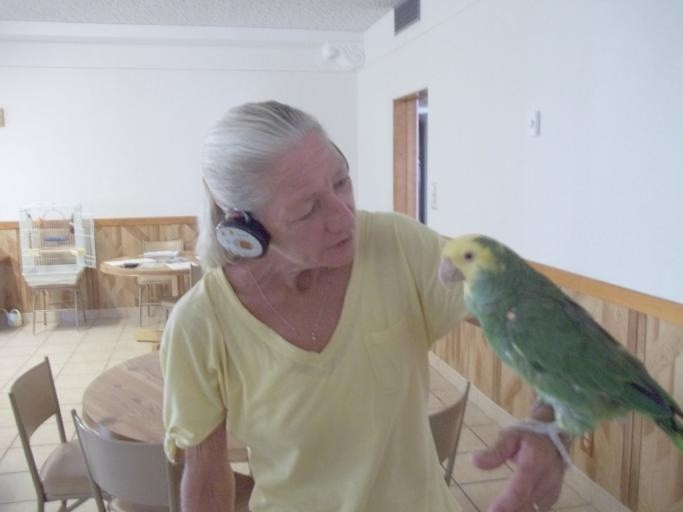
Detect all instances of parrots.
[438,234,683,473]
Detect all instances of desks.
[77,348,248,511]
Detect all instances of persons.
[155,100,582,512]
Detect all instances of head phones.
[214,140,349,261]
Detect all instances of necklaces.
[243,259,341,343]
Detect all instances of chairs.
[427,382,473,482]
[68,405,179,512]
[0,240,212,346]
[8,356,116,512]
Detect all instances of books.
[143,249,179,263]
